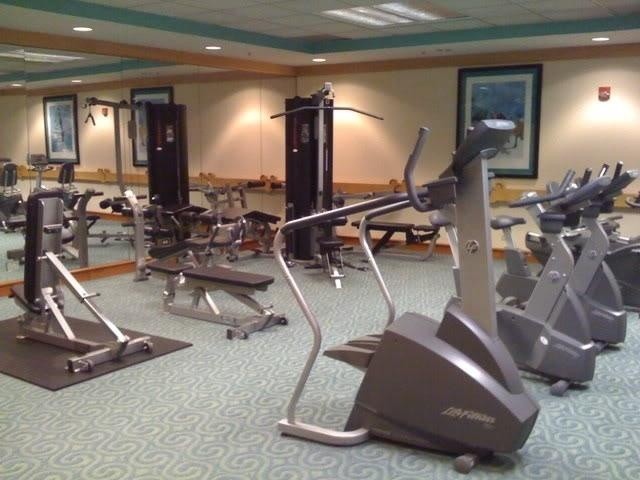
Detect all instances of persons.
[512,113,524,148]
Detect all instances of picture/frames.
[130,86,173,166]
[456,64,542,178]
[42,95,80,165]
